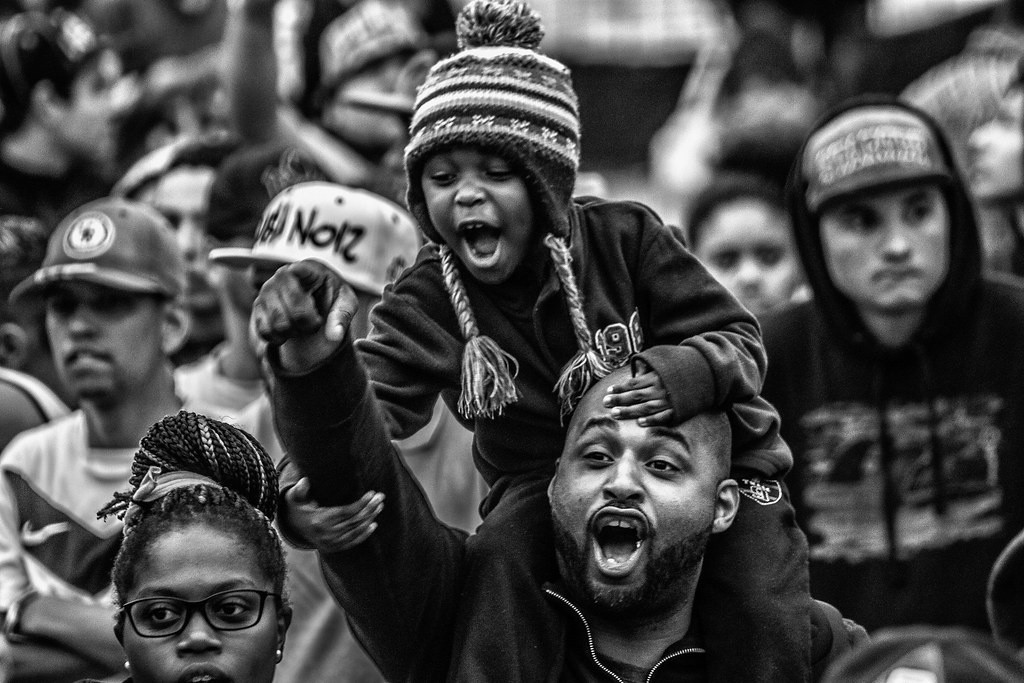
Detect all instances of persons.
[0,0,1024,683]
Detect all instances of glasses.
[116,589,280,639]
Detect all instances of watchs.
[3,590,37,644]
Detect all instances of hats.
[802,105,950,209]
[9,197,185,318]
[405,0,615,419]
[0,8,112,101]
[207,181,423,297]
